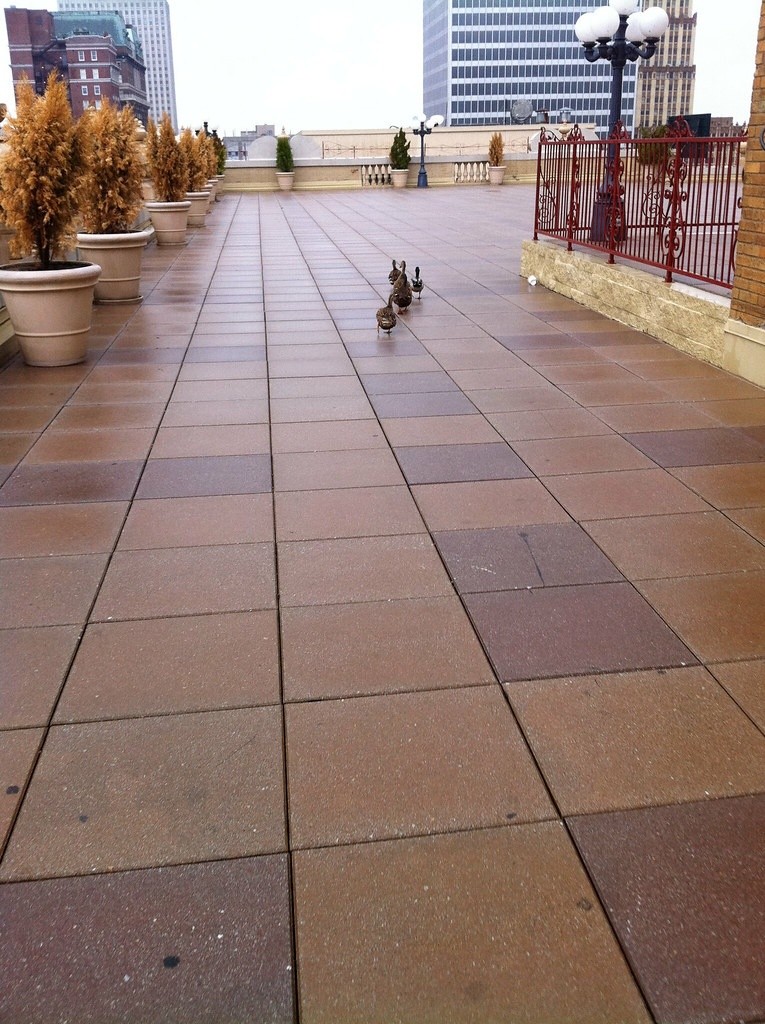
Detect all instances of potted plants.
[0,67,226,367]
[637,123,672,184]
[389,128,411,187]
[275,137,295,190]
[488,133,506,185]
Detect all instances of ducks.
[376,293,400,334]
[392,261,412,314]
[410,267,424,300]
[389,260,402,285]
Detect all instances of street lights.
[410,113,437,187]
[572,0,670,239]
